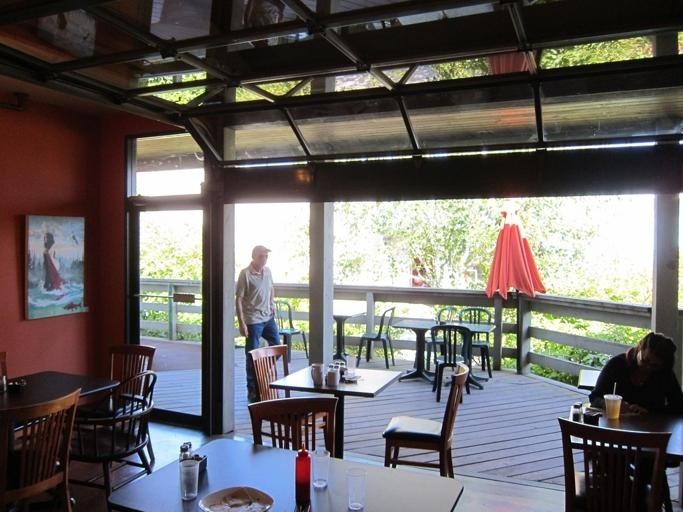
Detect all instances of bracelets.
[270,303,274,307]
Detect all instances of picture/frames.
[20,209,91,322]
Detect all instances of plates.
[197,486,274,512]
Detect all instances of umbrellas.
[484,202,547,301]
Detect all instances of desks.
[312,307,368,364]
[106,437,576,510]
[267,362,403,459]
[564,401,682,491]
[2,368,123,419]
[0,387,84,511]
[573,365,600,392]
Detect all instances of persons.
[588,332,683,415]
[236,245,281,402]
[43,232,65,290]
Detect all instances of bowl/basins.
[5,379,30,392]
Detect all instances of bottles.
[329,360,346,380]
[573,402,582,422]
[1,376,6,393]
[295,444,312,507]
[178,441,192,459]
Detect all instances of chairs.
[243,394,339,459]
[268,299,310,362]
[61,368,160,501]
[353,306,398,370]
[246,343,331,450]
[105,340,157,470]
[386,306,498,406]
[379,361,471,482]
[554,415,675,511]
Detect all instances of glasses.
[641,353,656,367]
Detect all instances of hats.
[252,245,271,254]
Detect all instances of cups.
[346,468,367,511]
[603,393,622,420]
[310,363,324,386]
[178,459,200,502]
[328,369,341,387]
[312,450,330,488]
